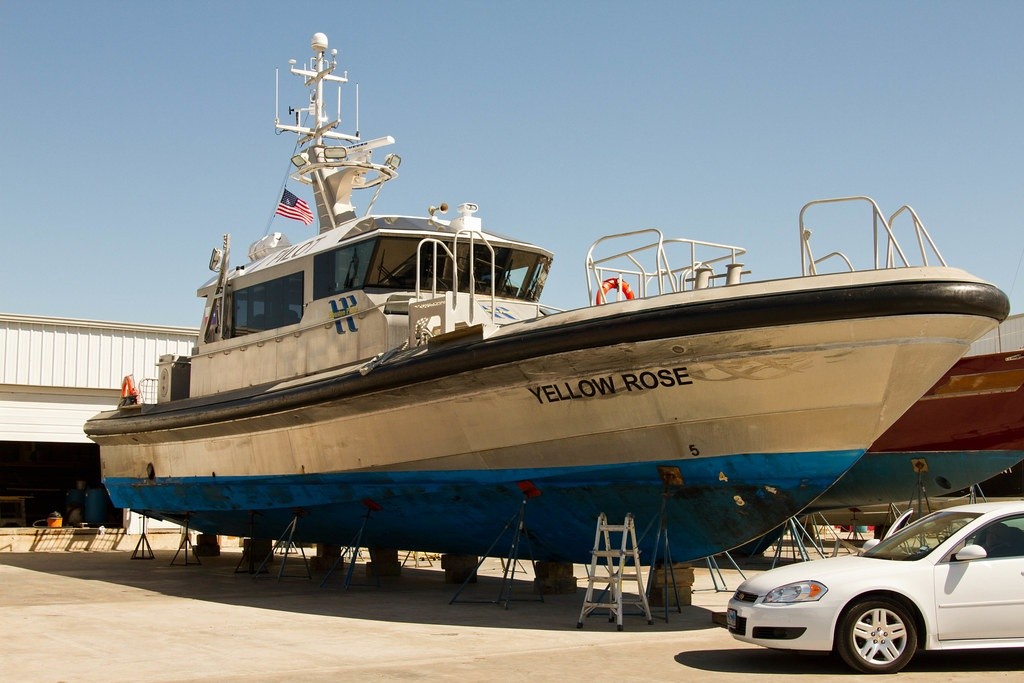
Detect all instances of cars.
[727,501,1023,675]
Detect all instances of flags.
[274,189,313,225]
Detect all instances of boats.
[714,313,1024,557]
[82,33,1011,568]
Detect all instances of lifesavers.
[122,376,133,398]
[596,278,636,304]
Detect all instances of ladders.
[576,513,653,631]
